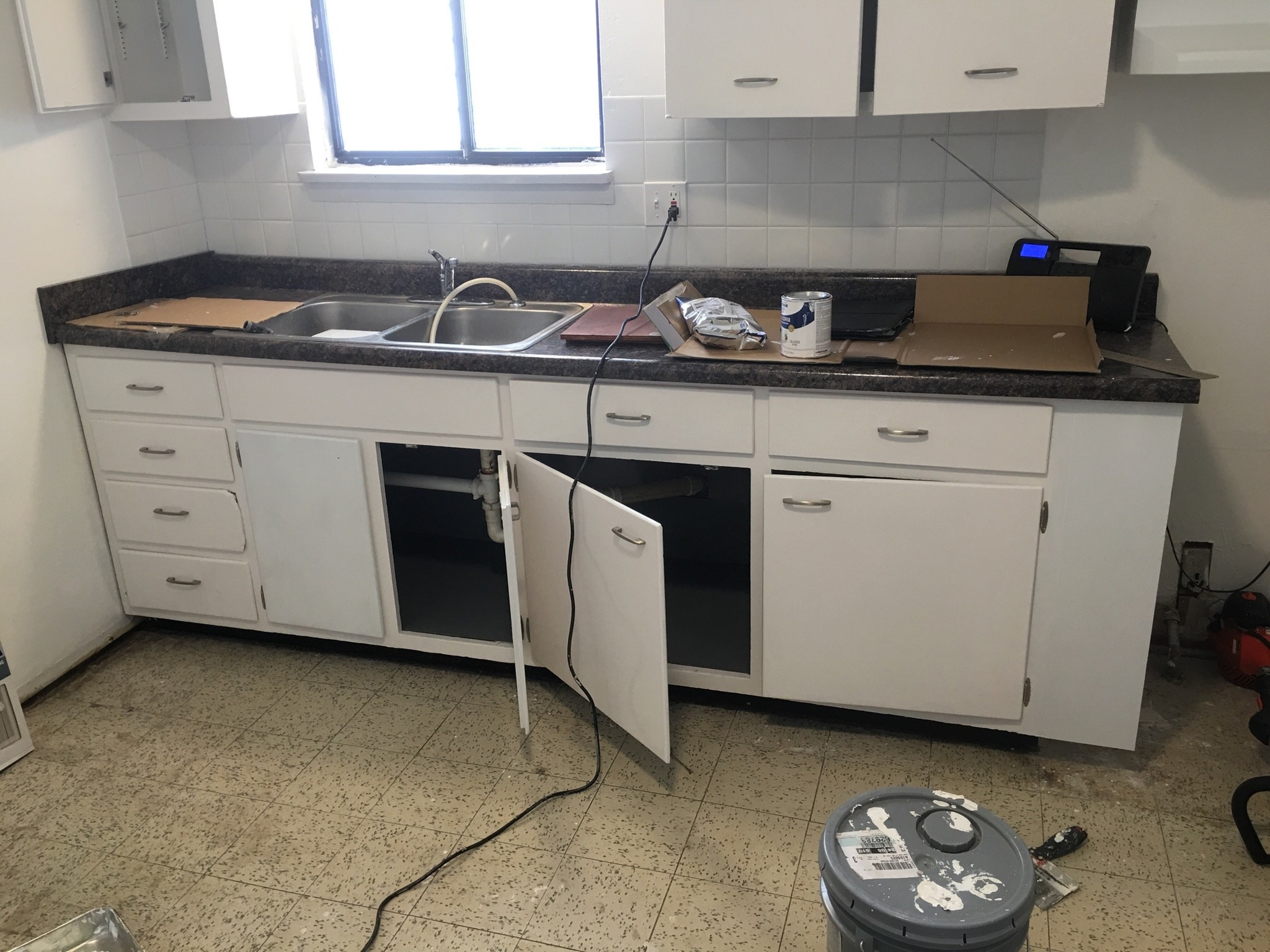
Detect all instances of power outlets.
[1181,548,1211,591]
[643,182,688,227]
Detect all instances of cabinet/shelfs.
[63,345,1184,766]
[17,0,301,122]
[665,0,1270,121]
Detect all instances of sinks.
[380,300,594,351]
[213,291,440,342]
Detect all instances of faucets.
[428,249,459,301]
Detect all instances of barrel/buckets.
[781,291,832,358]
[818,787,1036,952]
